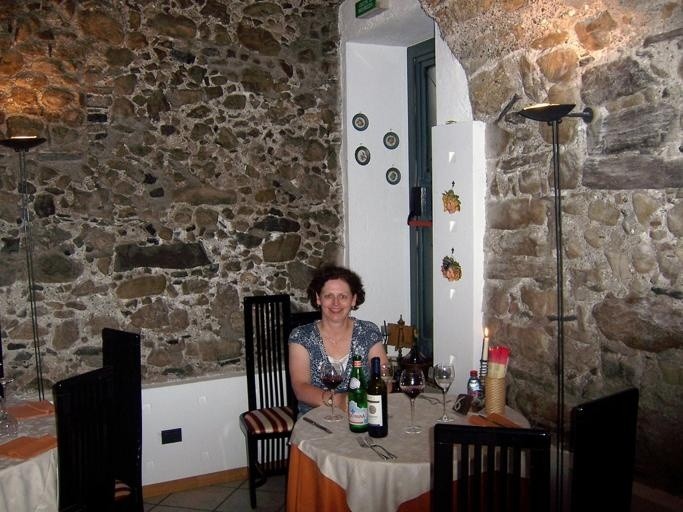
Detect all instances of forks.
[423,395,451,404]
[357,438,389,460]
[365,437,398,460]
[420,396,438,405]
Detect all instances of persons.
[289,266,392,422]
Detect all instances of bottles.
[367,356,387,438]
[349,356,368,432]
[403,334,429,382]
[466,370,480,400]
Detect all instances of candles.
[481,327,490,361]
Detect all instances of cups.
[380,363,394,418]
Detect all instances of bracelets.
[322,391,329,407]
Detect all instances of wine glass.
[400,368,425,434]
[0,378,18,438]
[435,361,456,422]
[321,362,345,420]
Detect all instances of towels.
[468,412,522,429]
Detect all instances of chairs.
[101,326,144,512]
[434,423,551,512]
[283,311,328,423]
[239,293,299,509]
[52,364,116,512]
[569,388,639,512]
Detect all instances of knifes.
[304,417,333,434]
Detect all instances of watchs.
[327,394,333,407]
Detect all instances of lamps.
[518,103,576,512]
[0,135,47,402]
